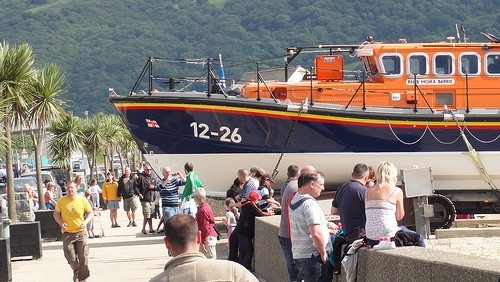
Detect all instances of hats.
[250,166,260,173]
[257,169,267,176]
[249,191,262,201]
[262,174,275,183]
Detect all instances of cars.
[0,166,107,202]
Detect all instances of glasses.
[146,169,151,171]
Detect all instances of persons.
[53,181,93,282]
[179,162,204,218]
[117,167,137,227]
[222,197,239,257]
[222,166,280,272]
[157,166,187,255]
[135,164,156,234]
[280,165,300,197]
[364,164,376,189]
[149,213,259,282]
[234,192,274,272]
[288,171,329,282]
[101,171,122,228]
[193,187,218,260]
[364,162,426,248]
[278,165,316,282]
[22,176,108,239]
[286,174,304,282]
[330,164,369,243]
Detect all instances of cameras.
[172,172,177,176]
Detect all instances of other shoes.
[93,235,100,238]
[88,237,93,238]
[73,271,79,282]
[112,224,117,228]
[149,230,155,233]
[115,223,121,227]
[132,221,137,226]
[127,221,133,227]
[142,230,147,234]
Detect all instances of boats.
[106,23,500,200]
